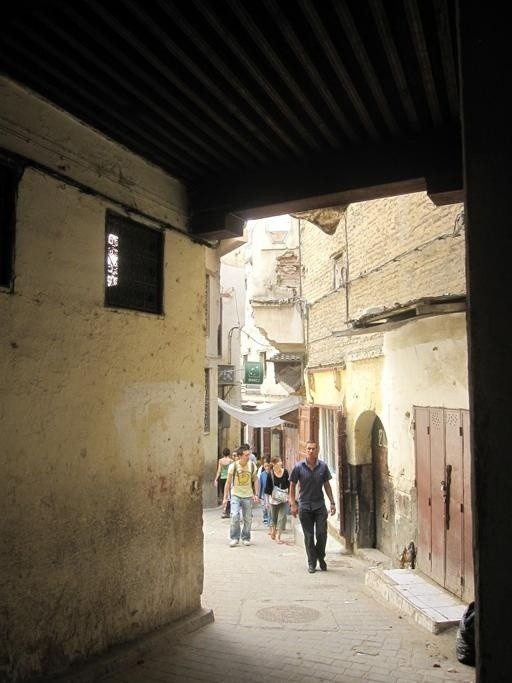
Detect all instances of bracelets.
[331,502,335,506]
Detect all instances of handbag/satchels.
[269,486,290,505]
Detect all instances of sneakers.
[308,562,317,574]
[220,511,231,520]
[242,538,252,548]
[228,539,240,548]
[318,558,328,571]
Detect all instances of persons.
[214,444,290,549]
[288,441,337,573]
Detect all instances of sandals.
[270,533,284,545]
